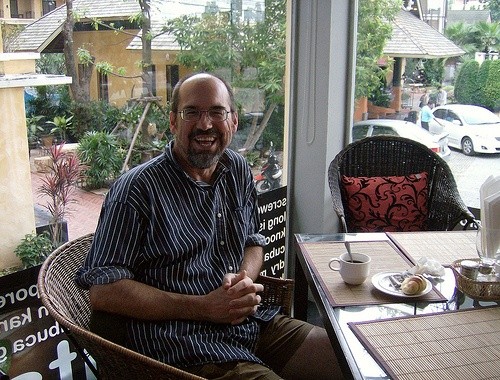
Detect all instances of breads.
[401,274,426,295]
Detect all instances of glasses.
[178,108,230,122]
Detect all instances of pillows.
[339,173,428,231]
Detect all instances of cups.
[475,224,500,264]
[328,252,371,285]
[460,260,479,280]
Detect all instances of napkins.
[479,175,500,230]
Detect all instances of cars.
[415,104,500,157]
[351,119,452,164]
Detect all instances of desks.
[56,143,83,156]
[290,231,500,380]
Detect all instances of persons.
[419,100,444,133]
[436,86,447,106]
[75,72,344,379]
[420,90,429,106]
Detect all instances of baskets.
[451,258,500,301]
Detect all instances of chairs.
[38,135,57,156]
[37,231,294,380]
[328,137,480,232]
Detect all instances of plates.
[371,271,432,298]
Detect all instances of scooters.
[255,140,283,193]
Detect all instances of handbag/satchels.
[419,101,422,107]
